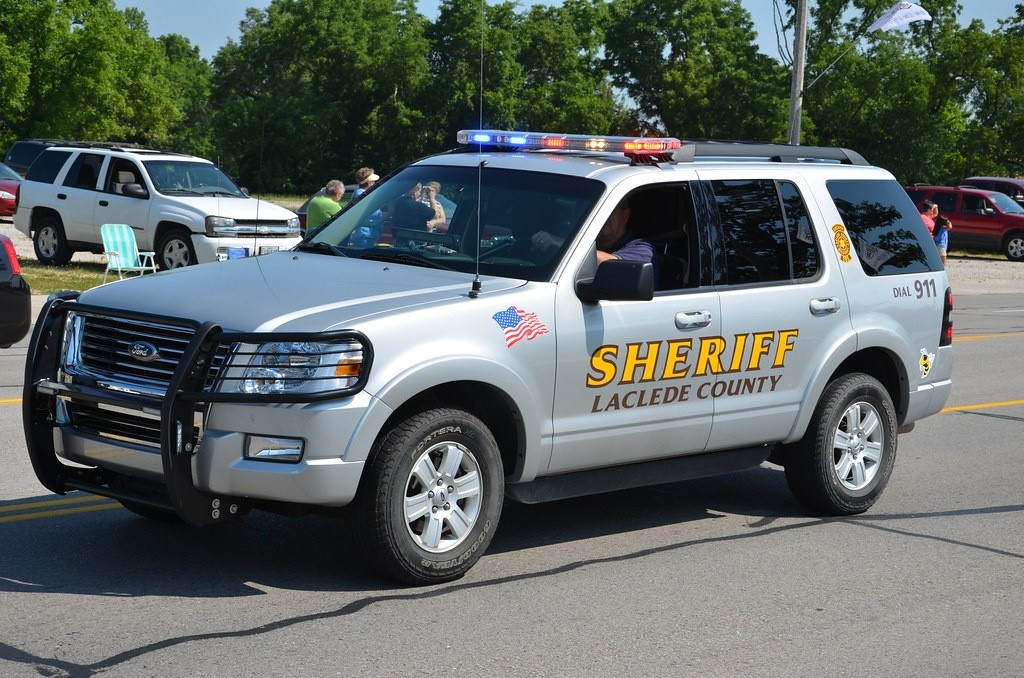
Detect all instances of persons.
[305,180,345,238]
[390,181,447,233]
[921,200,953,267]
[348,166,385,250]
[531,189,661,296]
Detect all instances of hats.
[359,170,380,184]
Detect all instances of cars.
[0,161,26,216]
[297,182,514,257]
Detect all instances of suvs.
[22,129,955,585]
[13,146,304,272]
[904,182,1024,262]
[4,138,154,179]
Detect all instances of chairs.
[458,191,531,260]
[112,170,137,193]
[100,222,158,284]
[642,204,691,289]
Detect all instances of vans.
[960,177,1024,206]
